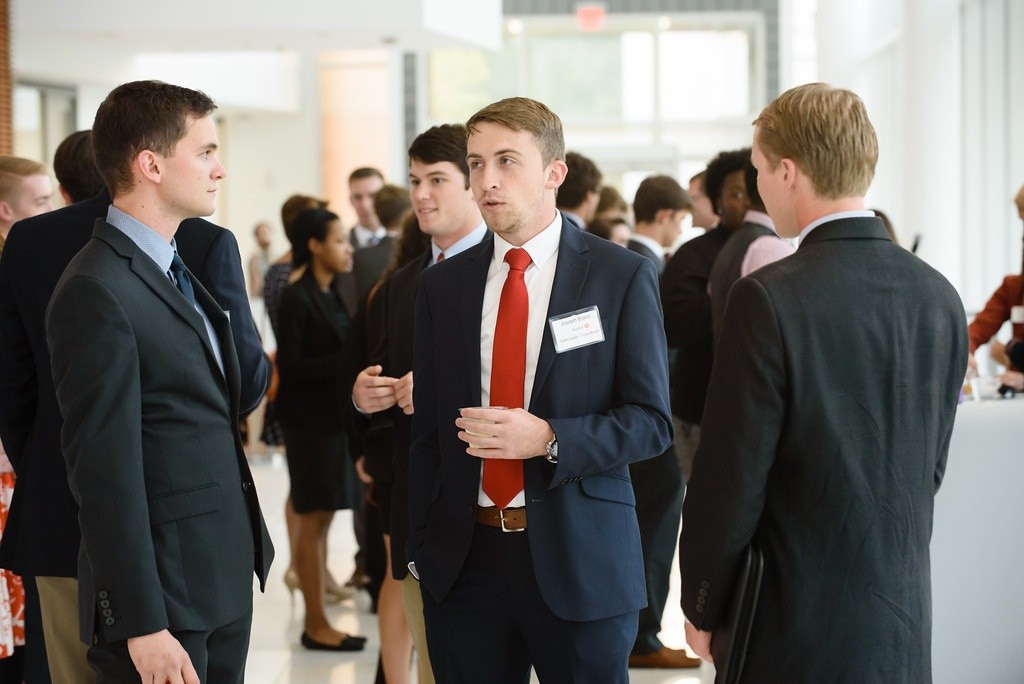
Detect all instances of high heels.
[286,563,352,602]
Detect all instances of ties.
[171,253,196,310]
[369,237,379,245]
[437,253,444,264]
[482,249,533,510]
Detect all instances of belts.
[477,506,528,533]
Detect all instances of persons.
[968,185,1024,393]
[407,95,676,684]
[252,124,487,684]
[556,147,795,668]
[46,82,275,684]
[1,132,269,683]
[678,84,969,684]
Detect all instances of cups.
[970,378,1003,400]
[458,406,509,449]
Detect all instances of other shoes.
[302,631,369,651]
[628,644,705,669]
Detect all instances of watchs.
[546,433,558,463]
